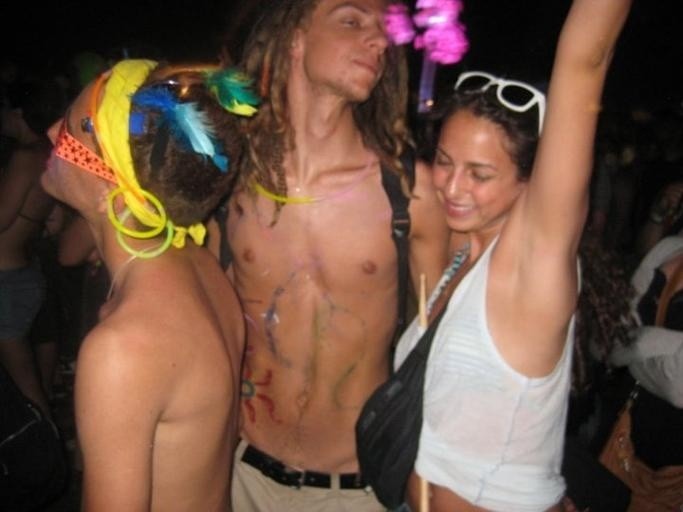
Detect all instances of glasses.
[56,104,122,188]
[453,71,547,139]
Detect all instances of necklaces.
[248,154,375,202]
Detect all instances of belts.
[236,440,368,490]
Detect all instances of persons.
[541,93,682,511]
[392,0,632,512]
[206,2,451,511]
[2,57,110,512]
[39,59,263,512]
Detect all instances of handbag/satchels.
[599,406,683,511]
[356,328,431,508]
